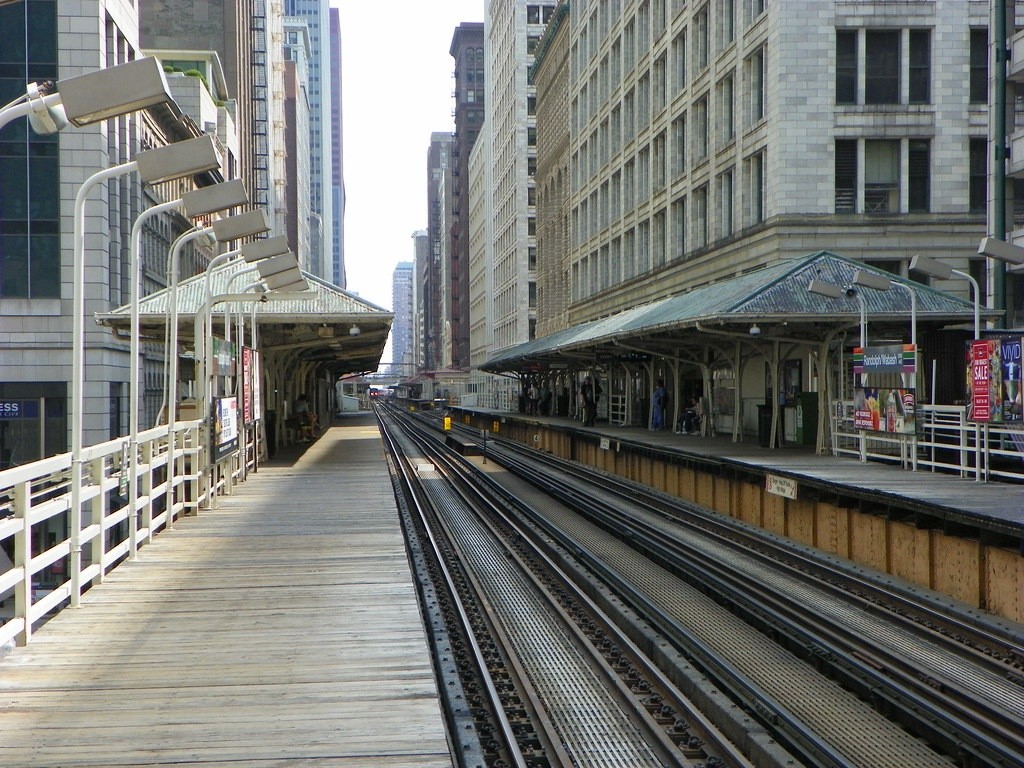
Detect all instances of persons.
[650,378,666,431]
[677,396,701,436]
[293,393,324,443]
[581,376,603,427]
[526,381,550,418]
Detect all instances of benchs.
[279,427,296,446]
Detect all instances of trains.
[370,387,394,399]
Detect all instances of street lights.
[203,235,310,513]
[68,135,224,610]
[852,270,918,472]
[909,254,982,484]
[129,176,251,561]
[807,279,868,464]
[164,207,272,531]
